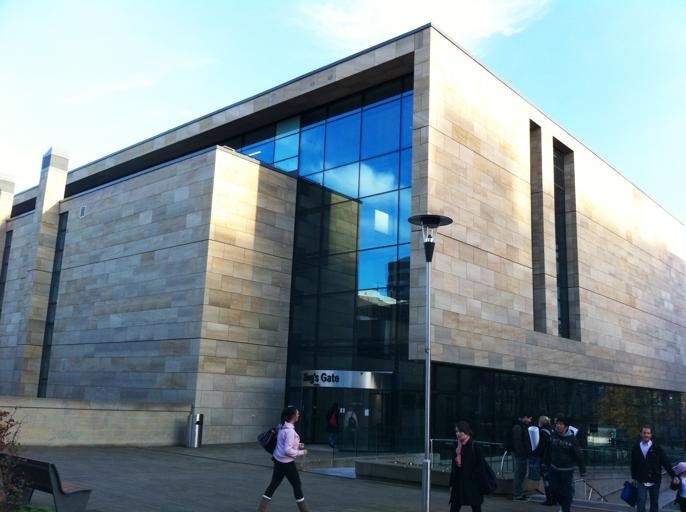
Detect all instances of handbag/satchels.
[471,440,497,495]
[257,428,278,455]
[621,479,637,507]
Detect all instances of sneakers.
[512,495,529,502]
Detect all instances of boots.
[540,487,552,506]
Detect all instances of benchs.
[0,451,91,512]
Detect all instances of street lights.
[406,207,453,511]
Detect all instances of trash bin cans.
[186,413,203,448]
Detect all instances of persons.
[510,412,533,502]
[327,402,340,447]
[344,405,359,449]
[258,406,310,512]
[548,418,586,512]
[630,425,679,512]
[538,416,554,506]
[669,462,686,512]
[448,420,488,512]
